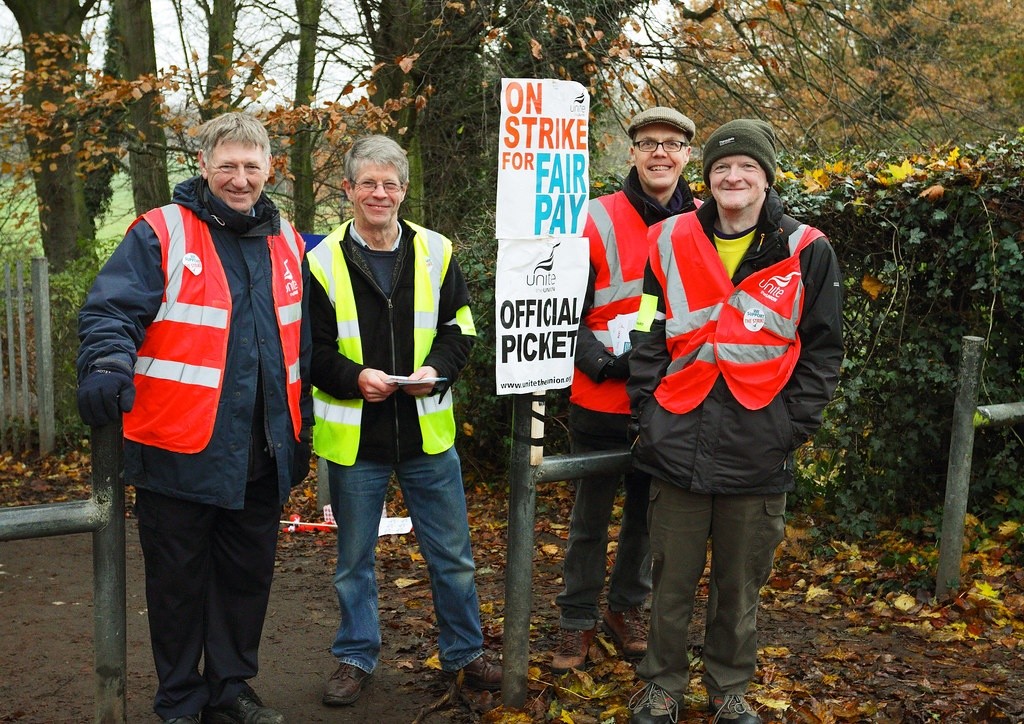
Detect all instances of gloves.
[606,350,636,381]
[75,363,136,427]
[290,423,311,487]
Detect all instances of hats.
[702,119,777,188]
[627,107,695,142]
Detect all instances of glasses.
[350,177,405,193]
[633,140,688,153]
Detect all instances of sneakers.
[551,621,597,674]
[440,655,502,689]
[599,607,648,660]
[322,663,374,705]
[162,713,200,724]
[706,691,762,724]
[199,690,286,724]
[629,683,679,724]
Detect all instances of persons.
[304,134,501,704]
[75,114,315,724]
[626,119,845,724]
[550,107,705,675]
